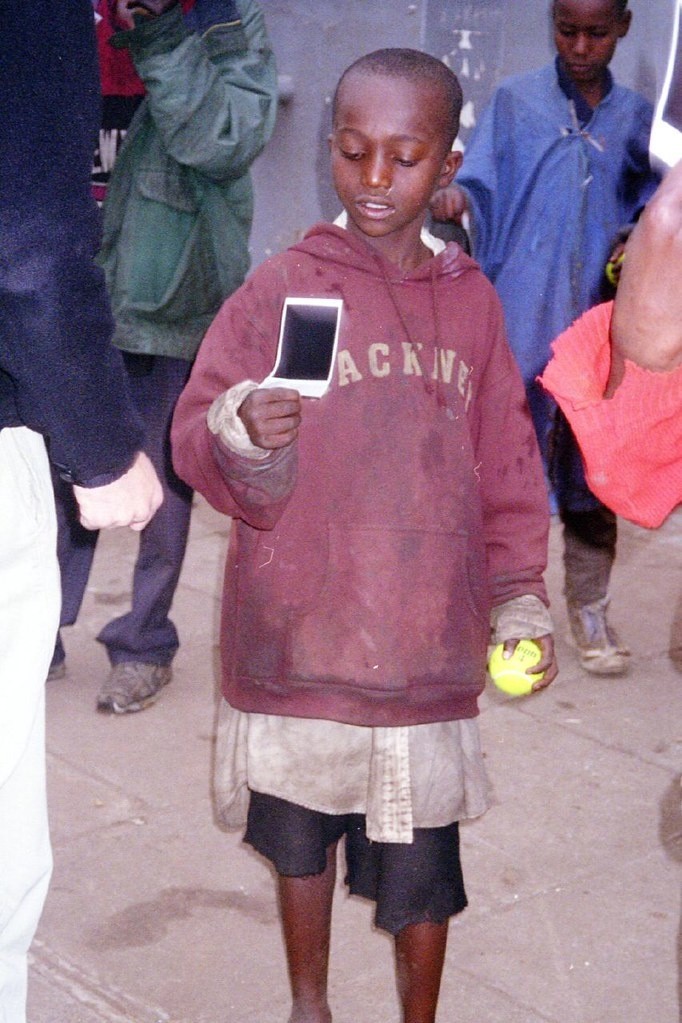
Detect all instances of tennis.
[488,639,547,698]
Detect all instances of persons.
[0,0,158,1023]
[171,48,560,1022]
[425,0,682,678]
[45,0,278,715]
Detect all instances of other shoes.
[567,597,628,673]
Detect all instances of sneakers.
[96,662,171,714]
[46,663,66,682]
